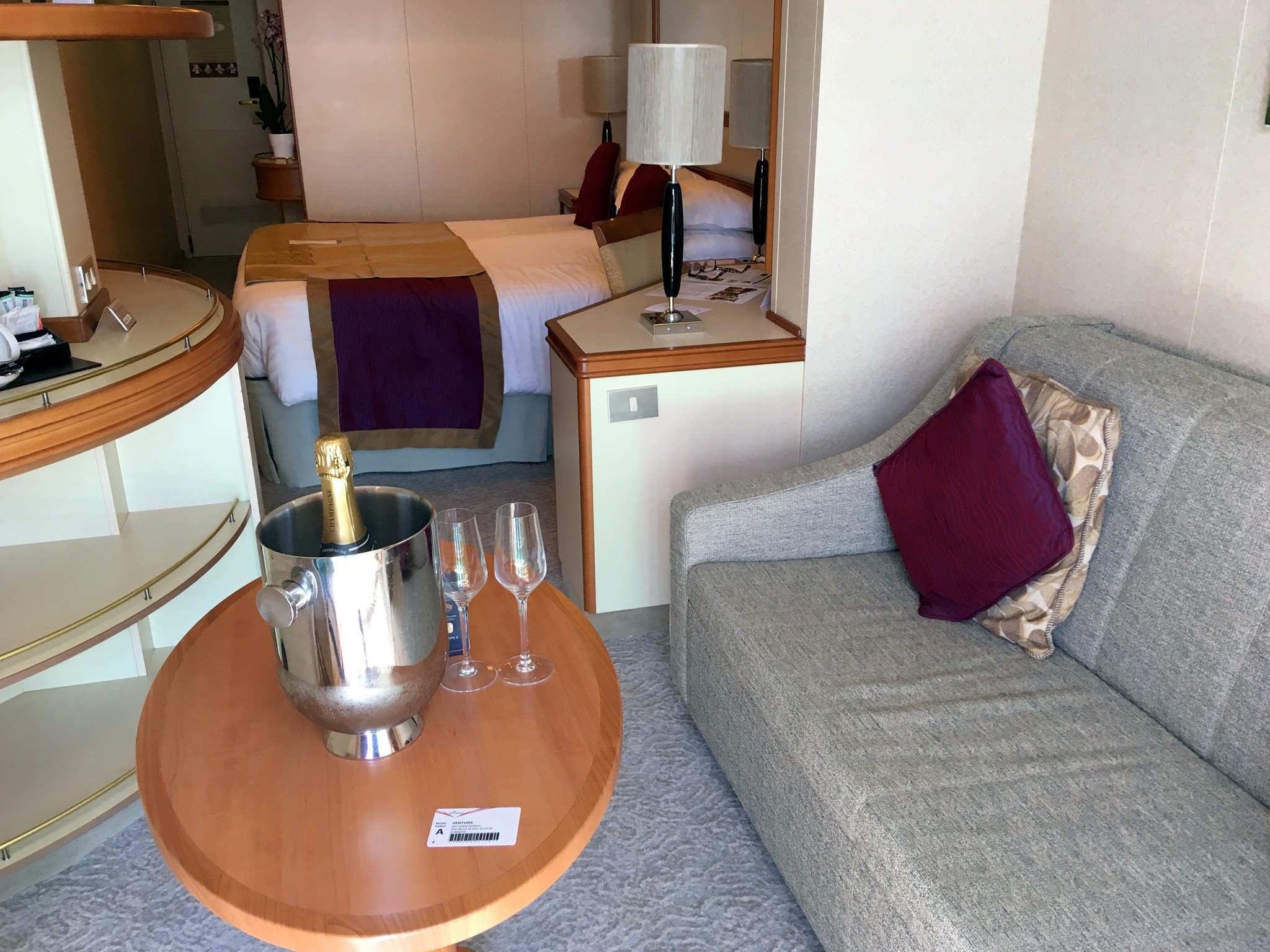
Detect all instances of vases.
[268,133,295,158]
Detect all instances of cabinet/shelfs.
[0,258,268,905]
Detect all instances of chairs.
[592,206,663,297]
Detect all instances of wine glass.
[432,508,498,694]
[494,502,554,685]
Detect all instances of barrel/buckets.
[255,485,439,762]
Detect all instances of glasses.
[688,259,751,282]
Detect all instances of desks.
[682,259,742,274]
[134,535,622,952]
[252,152,304,223]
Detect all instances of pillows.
[574,142,621,230]
[873,357,1075,621]
[947,347,1122,659]
[613,161,753,232]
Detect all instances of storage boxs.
[13,324,72,373]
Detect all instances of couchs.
[668,314,1270,952]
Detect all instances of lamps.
[625,42,727,336]
[730,59,772,263]
[582,54,627,142]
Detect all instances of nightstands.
[558,187,581,215]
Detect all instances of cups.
[0,322,21,362]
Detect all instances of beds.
[230,166,753,488]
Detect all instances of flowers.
[250,7,292,134]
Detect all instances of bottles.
[314,432,375,557]
